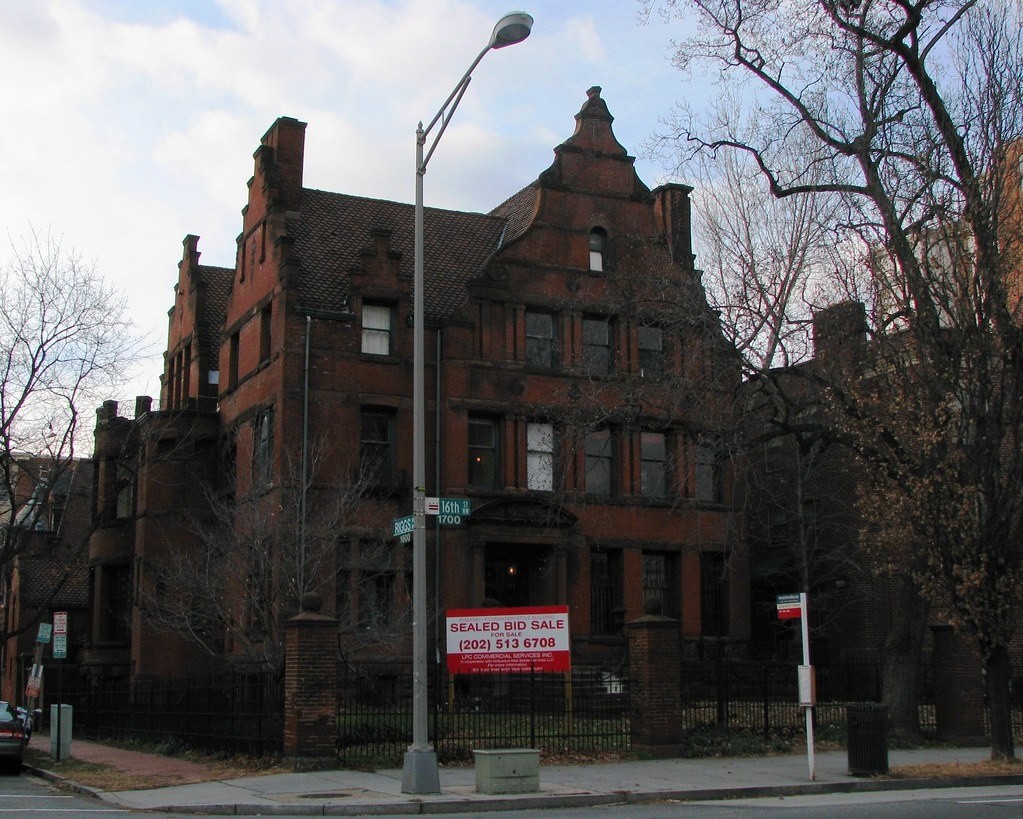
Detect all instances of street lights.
[398,8,540,795]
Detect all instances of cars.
[11,705,41,745]
[0,701,26,776]
